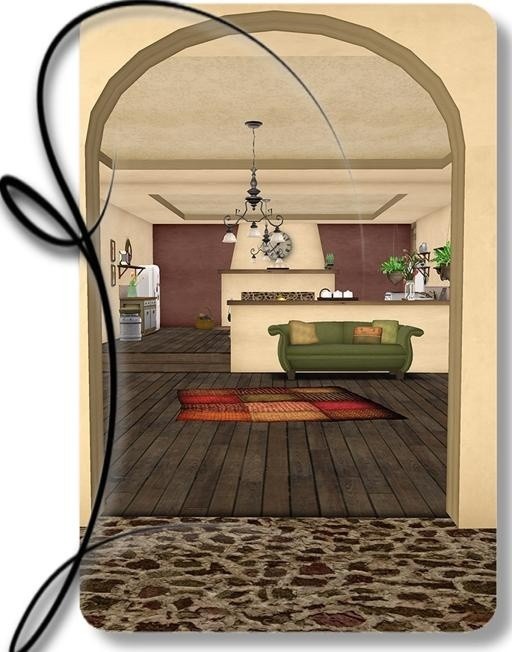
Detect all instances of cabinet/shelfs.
[118,296,157,337]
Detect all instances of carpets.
[177,385,409,422]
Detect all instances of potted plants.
[429,237,450,283]
[325,252,336,269]
[380,256,411,284]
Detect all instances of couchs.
[267,319,425,380]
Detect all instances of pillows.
[289,320,319,345]
[352,320,398,345]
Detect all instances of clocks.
[264,231,292,260]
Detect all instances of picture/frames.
[109,238,116,287]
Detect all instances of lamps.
[222,120,285,265]
